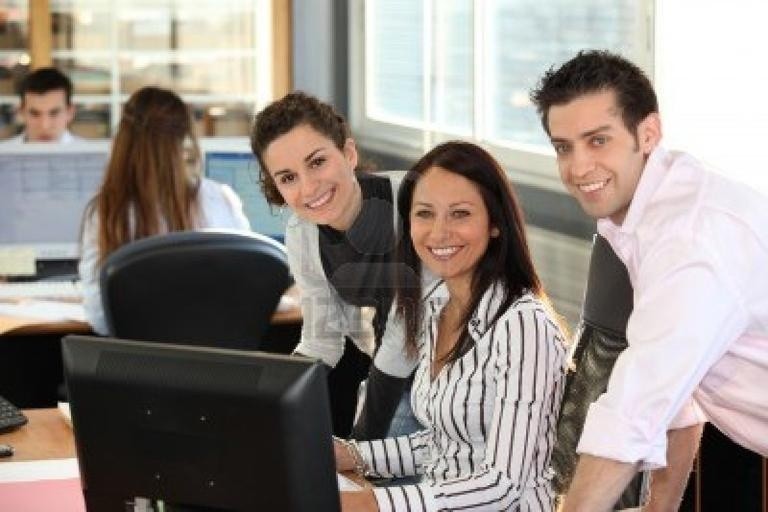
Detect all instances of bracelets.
[343,436,365,476]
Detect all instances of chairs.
[97,228,292,353]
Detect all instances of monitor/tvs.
[201,148,293,242]
[62,335,342,512]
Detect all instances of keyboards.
[0,396,28,430]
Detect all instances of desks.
[0,277,302,335]
[0,406,86,511]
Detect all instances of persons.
[247,90,435,441]
[334,138,573,511]
[78,83,255,338]
[1,65,97,147]
[529,49,767,510]
